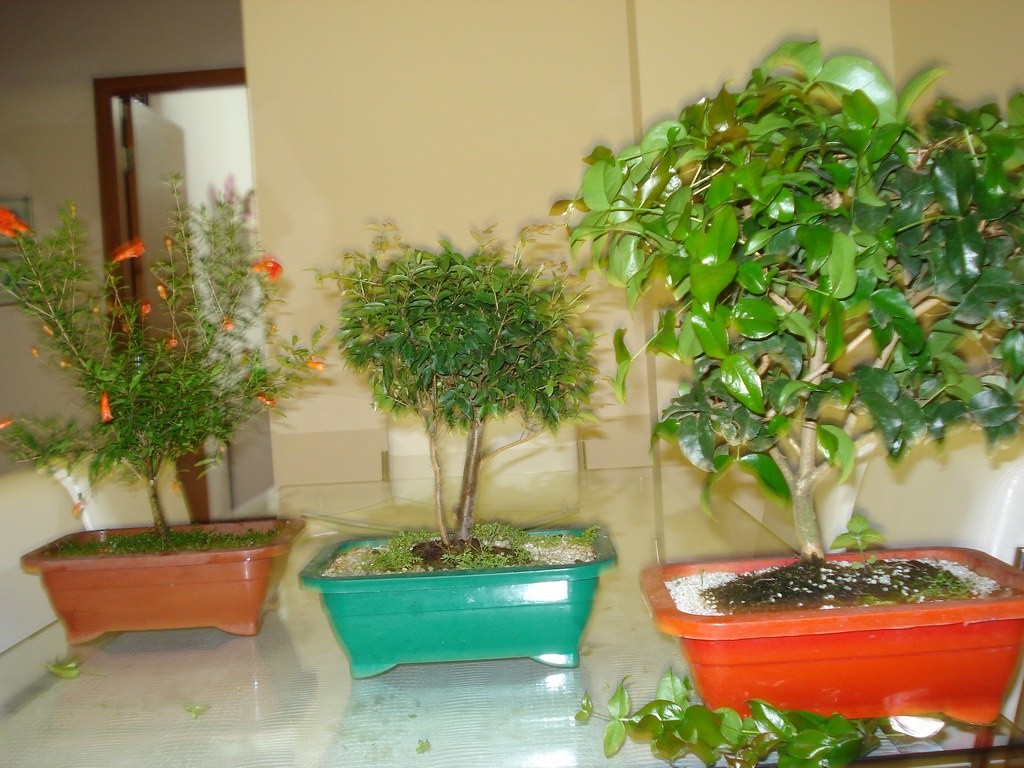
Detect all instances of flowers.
[0,159,330,534]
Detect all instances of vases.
[20,519,307,647]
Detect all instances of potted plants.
[550,39,1024,725]
[299,218,621,681]
